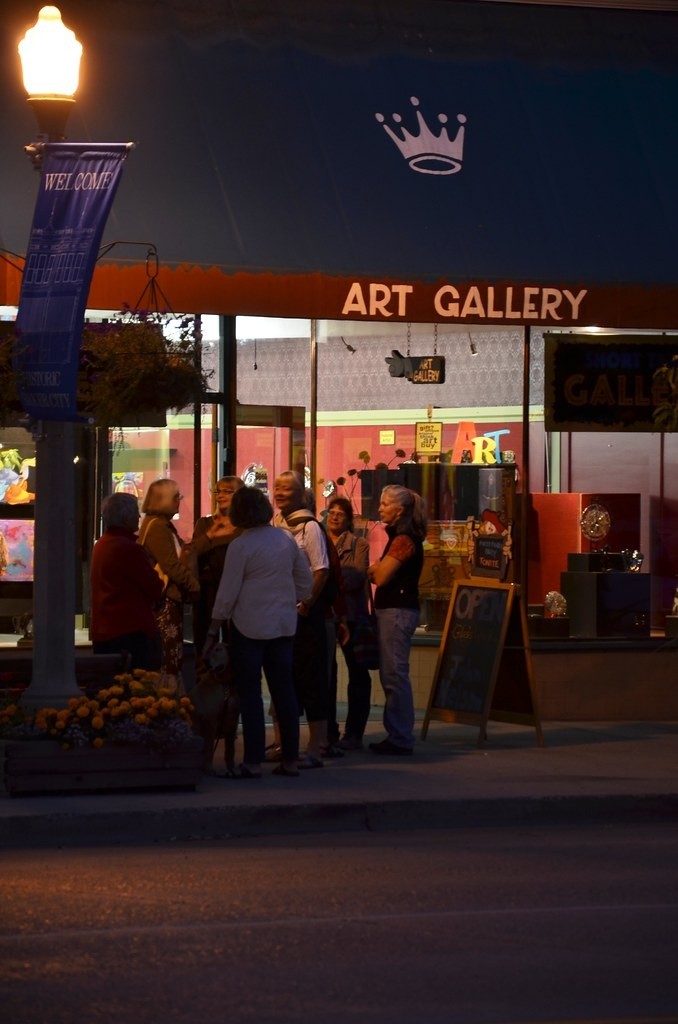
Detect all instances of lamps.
[347,345,356,354]
[470,344,478,354]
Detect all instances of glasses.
[175,495,184,500]
[214,490,234,496]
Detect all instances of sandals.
[319,744,345,758]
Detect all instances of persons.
[187,475,249,774]
[199,486,315,779]
[136,478,186,684]
[259,468,347,762]
[366,484,429,758]
[321,497,374,752]
[86,492,164,684]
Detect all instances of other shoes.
[337,733,363,749]
[265,744,300,763]
[369,738,414,756]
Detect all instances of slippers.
[295,754,323,769]
[270,763,299,777]
[216,763,253,779]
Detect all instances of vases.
[0,737,213,797]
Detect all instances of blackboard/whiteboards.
[424,578,519,726]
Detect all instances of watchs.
[206,631,220,643]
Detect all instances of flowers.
[0,327,37,411]
[0,669,199,748]
[83,302,213,427]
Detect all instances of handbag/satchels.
[364,615,383,671]
[168,583,190,605]
[190,640,240,743]
